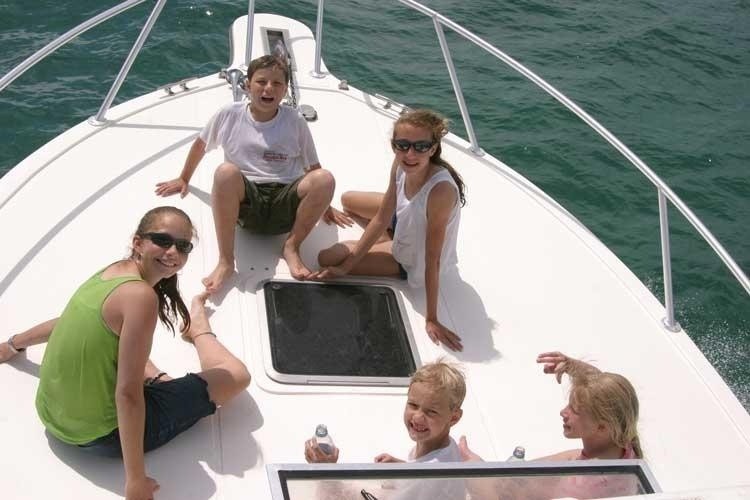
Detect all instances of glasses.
[139,232,195,254]
[389,138,437,154]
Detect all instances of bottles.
[505,446,525,462]
[311,424,334,455]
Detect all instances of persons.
[456,347,646,499]
[150,52,358,296]
[303,105,467,354]
[1,204,253,500]
[301,358,471,500]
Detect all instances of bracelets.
[5,332,26,357]
